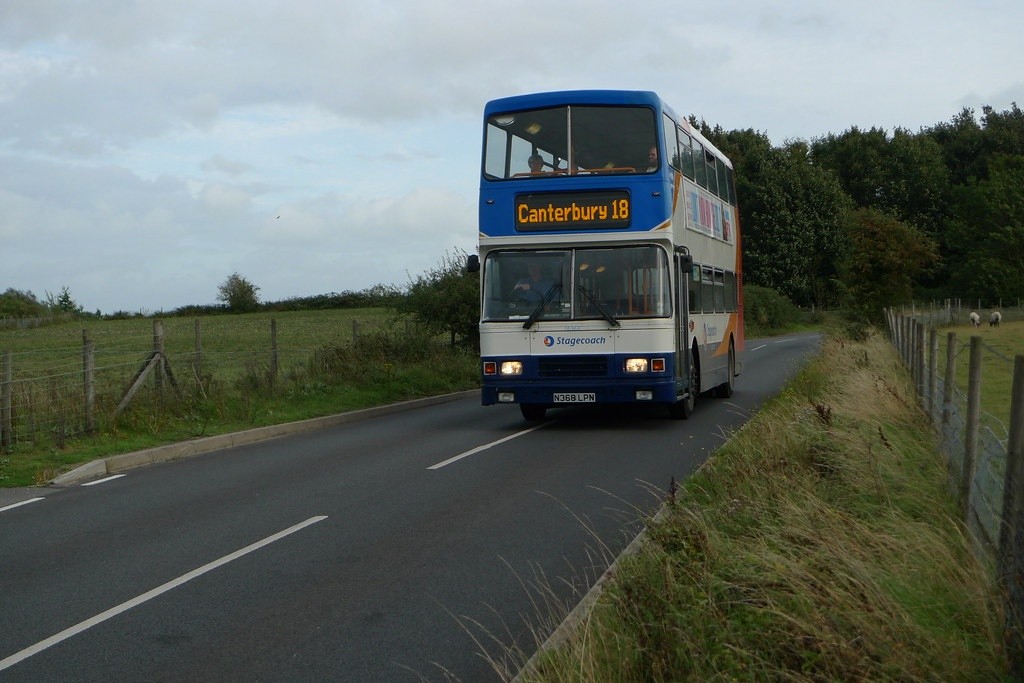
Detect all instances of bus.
[463,89,746,418]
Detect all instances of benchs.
[587,291,720,315]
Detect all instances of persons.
[528,154,545,172]
[638,146,657,172]
[509,260,552,306]
